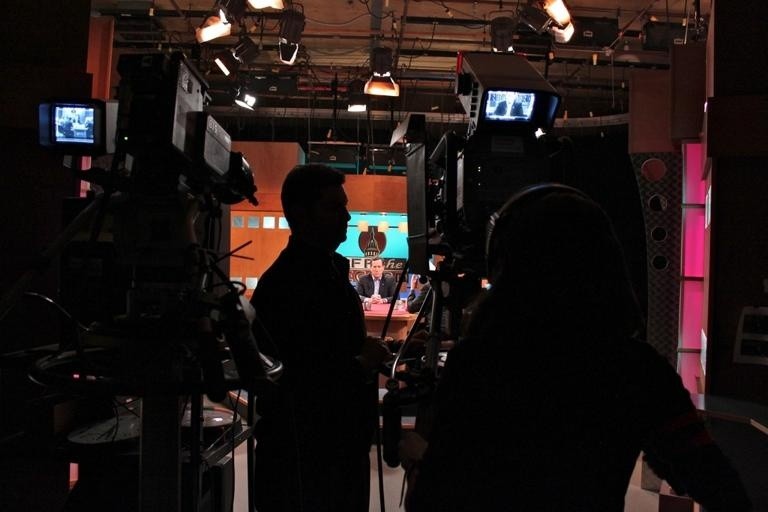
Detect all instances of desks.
[362,298,417,387]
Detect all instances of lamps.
[243,0,286,13]
[192,1,234,44]
[211,38,259,79]
[363,46,399,98]
[231,76,263,113]
[274,14,306,65]
[346,78,367,113]
[484,10,518,52]
[539,0,575,45]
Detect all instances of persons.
[494,94,524,116]
[398,185,755,512]
[357,258,400,304]
[407,261,437,313]
[249,164,391,512]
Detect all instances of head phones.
[481,184,589,297]
